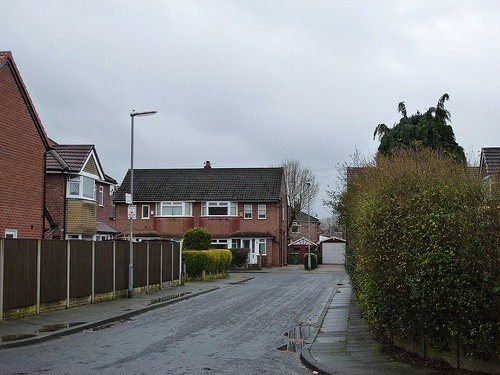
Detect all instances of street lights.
[127,109,156,299]
[306,181,311,271]
